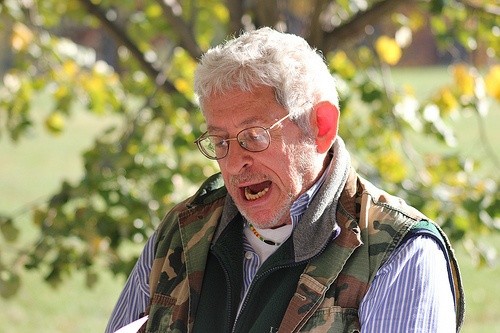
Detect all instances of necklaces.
[246,221,281,247]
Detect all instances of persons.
[104,25,466,333]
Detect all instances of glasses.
[194,98,314,160]
